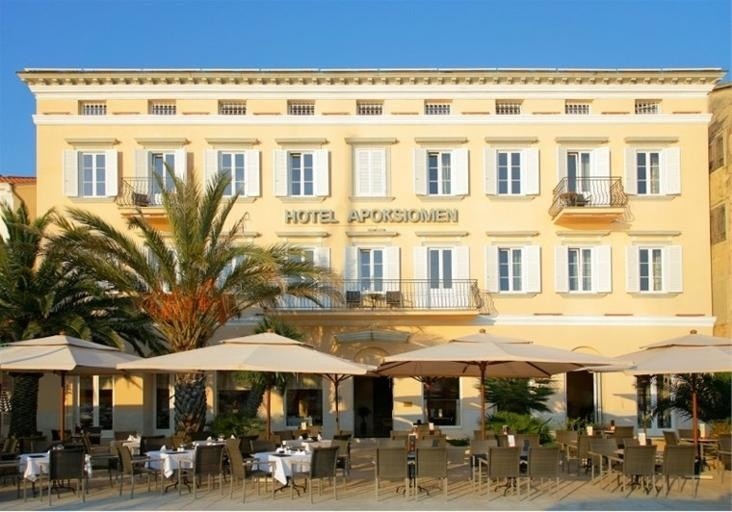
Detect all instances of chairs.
[342,288,407,311]
[1,426,225,506]
[186,423,354,504]
[679,427,732,485]
[468,429,563,499]
[556,424,697,499]
[374,424,448,501]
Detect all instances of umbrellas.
[588,330,732,482]
[0,332,141,445]
[314,353,381,436]
[375,328,637,439]
[113,329,364,443]
